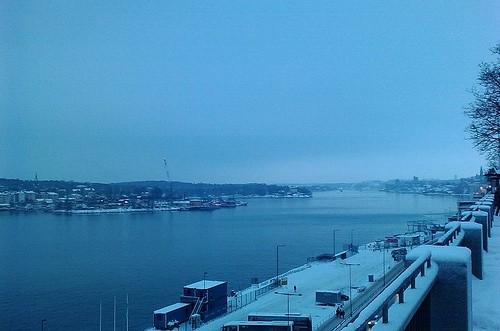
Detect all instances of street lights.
[350,228,355,248]
[333,229,341,256]
[203,272,208,290]
[339,262,360,317]
[275,291,303,331]
[277,245,286,287]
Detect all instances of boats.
[178,195,249,212]
